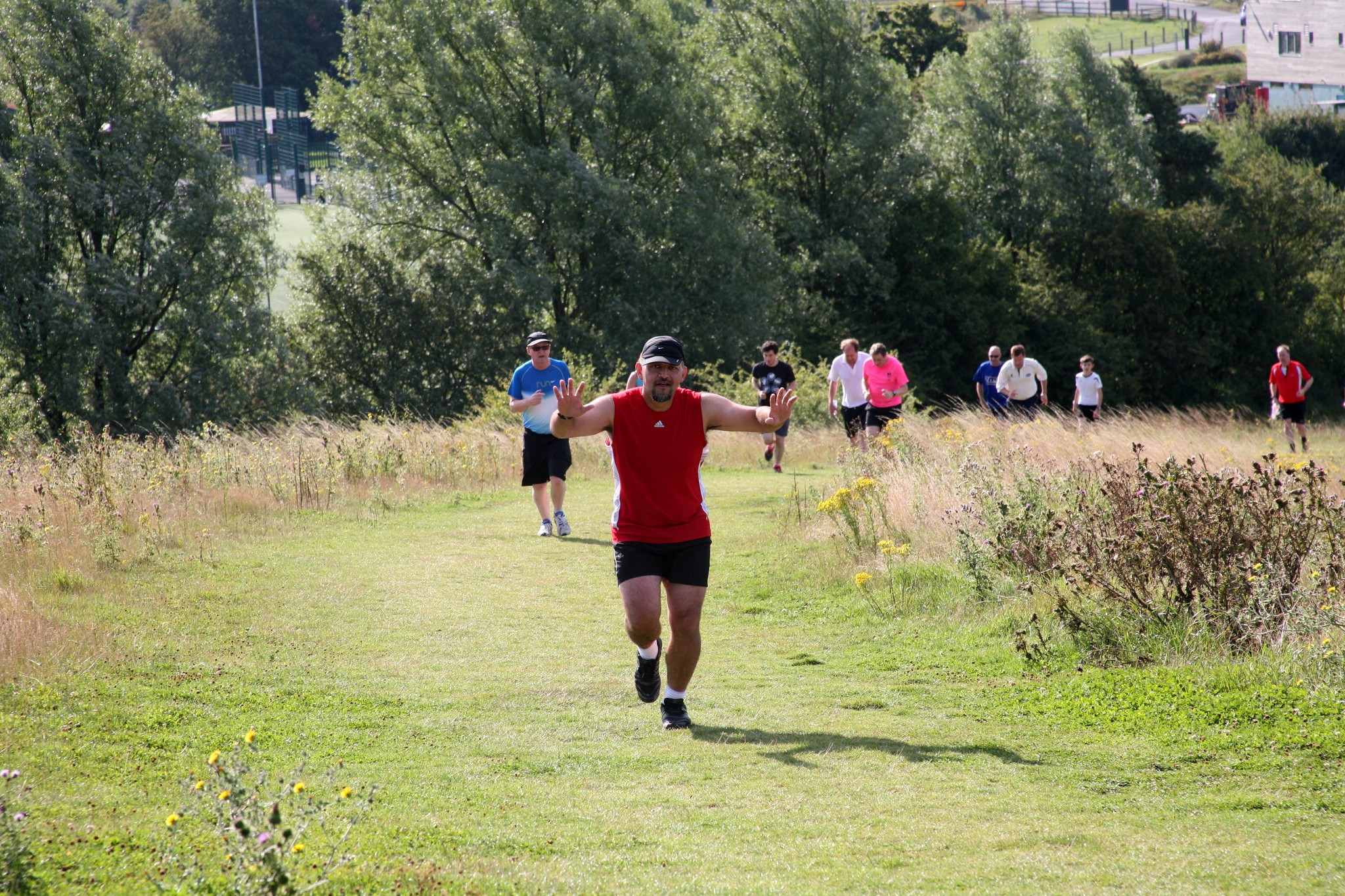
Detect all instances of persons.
[550,336,797,729]
[626,370,643,390]
[1072,355,1102,422]
[1269,344,1314,453]
[827,338,871,482]
[973,345,1012,419]
[996,344,1047,421]
[1245,89,1258,132]
[861,343,909,479]
[507,332,572,536]
[752,340,796,473]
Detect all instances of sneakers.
[554,514,571,536]
[537,522,552,536]
[764,443,775,461]
[773,464,782,473]
[635,638,662,703]
[661,698,691,730]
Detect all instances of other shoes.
[1302,444,1309,452]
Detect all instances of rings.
[783,401,787,405]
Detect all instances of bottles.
[833,400,838,415]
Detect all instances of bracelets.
[557,412,574,420]
[1271,396,1276,398]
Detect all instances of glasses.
[989,355,999,358]
[529,344,550,351]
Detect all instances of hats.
[526,332,550,347]
[639,335,684,366]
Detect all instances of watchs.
[892,391,896,396]
[1096,406,1101,410]
